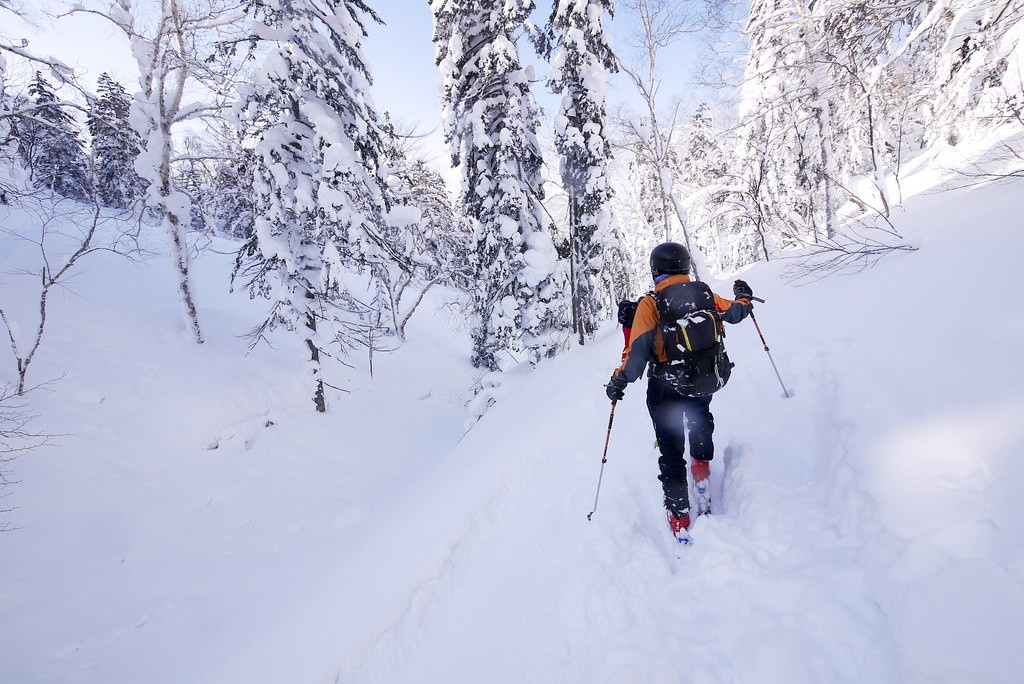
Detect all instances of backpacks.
[618,300,638,324]
[648,280,735,397]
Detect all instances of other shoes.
[666,509,691,539]
[690,457,710,492]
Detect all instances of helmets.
[650,242,691,275]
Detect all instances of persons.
[606,241,753,535]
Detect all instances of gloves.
[733,281,765,303]
[604,376,627,400]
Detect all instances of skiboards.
[664,486,713,549]
